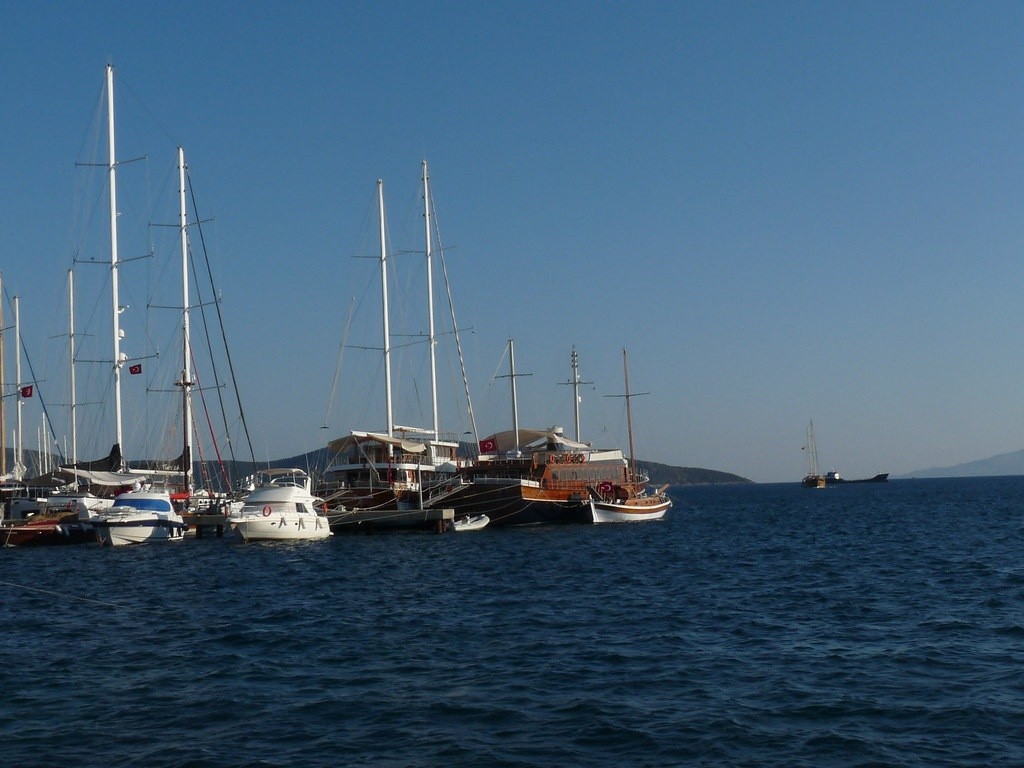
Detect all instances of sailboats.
[802,419,825,489]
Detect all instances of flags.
[22,385,33,398]
[388,462,393,487]
[597,482,614,494]
[129,363,142,374]
[479,434,499,452]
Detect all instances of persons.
[322,502,328,516]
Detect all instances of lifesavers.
[566,455,570,463]
[577,453,585,462]
[549,454,555,464]
[262,505,272,517]
[323,503,328,513]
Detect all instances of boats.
[0,64,626,547]
[590,484,671,525]
[519,346,650,503]
[826,467,889,482]
